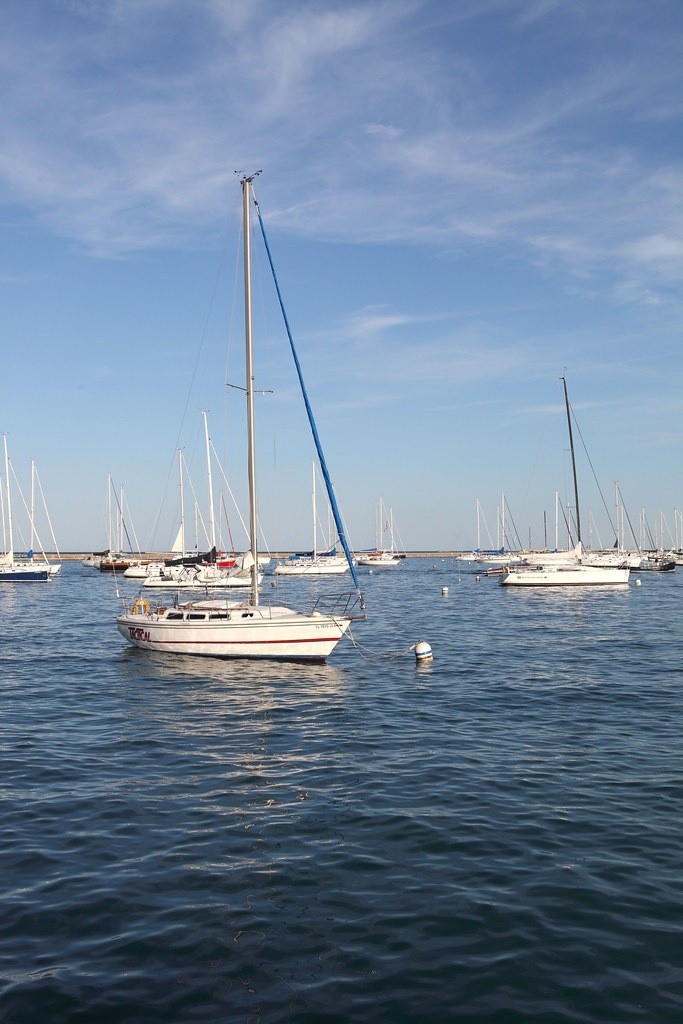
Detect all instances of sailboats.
[117,175,367,654]
[81,447,405,578]
[142,411,264,592]
[0,434,61,574]
[454,480,683,576]
[0,432,48,582]
[499,377,629,585]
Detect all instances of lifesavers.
[133,600,151,616]
